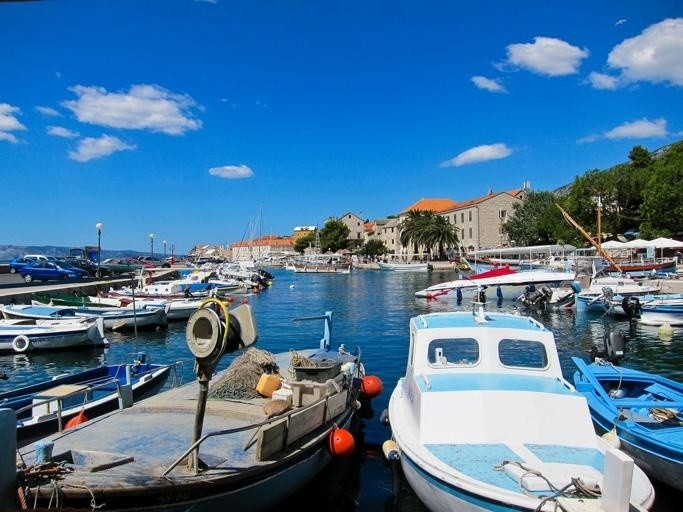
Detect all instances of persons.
[352,247,458,263]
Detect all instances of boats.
[234,201,380,275]
[419,299,574,327]
[413,197,682,328]
[568,314,682,346]
[12,297,383,512]
[569,352,683,495]
[380,306,656,511]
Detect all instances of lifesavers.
[11,335,30,353]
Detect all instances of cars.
[9,244,177,283]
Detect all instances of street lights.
[148,233,153,265]
[95,222,102,278]
[162,239,167,264]
